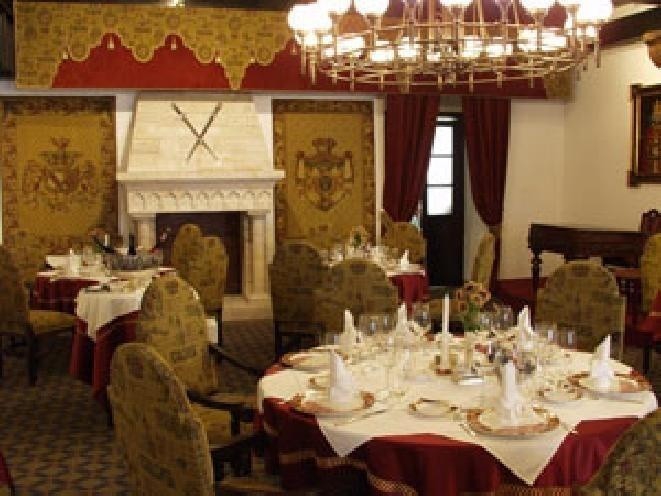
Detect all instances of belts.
[59,233,168,293]
[320,237,424,274]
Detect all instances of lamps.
[282,0,617,96]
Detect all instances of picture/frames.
[624,81,661,190]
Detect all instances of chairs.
[1,207,661,496]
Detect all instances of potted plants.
[624,81,661,190]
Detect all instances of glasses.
[282,0,617,96]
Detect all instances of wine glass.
[320,237,424,274]
[59,233,168,293]
[280,302,651,440]
